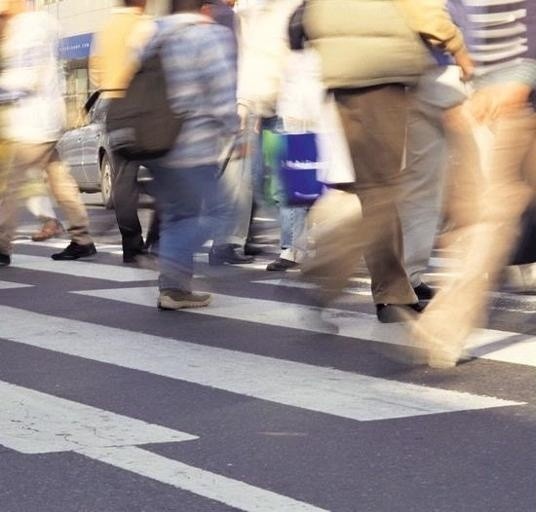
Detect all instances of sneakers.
[410,281,441,302]
[268,259,298,271]
[157,287,213,311]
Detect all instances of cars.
[54,87,157,211]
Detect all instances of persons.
[0,0,97,265]
[88,1,536,368]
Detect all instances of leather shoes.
[32,219,63,241]
[123,233,159,264]
[208,246,264,267]
[52,241,98,259]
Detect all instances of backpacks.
[107,21,220,162]
